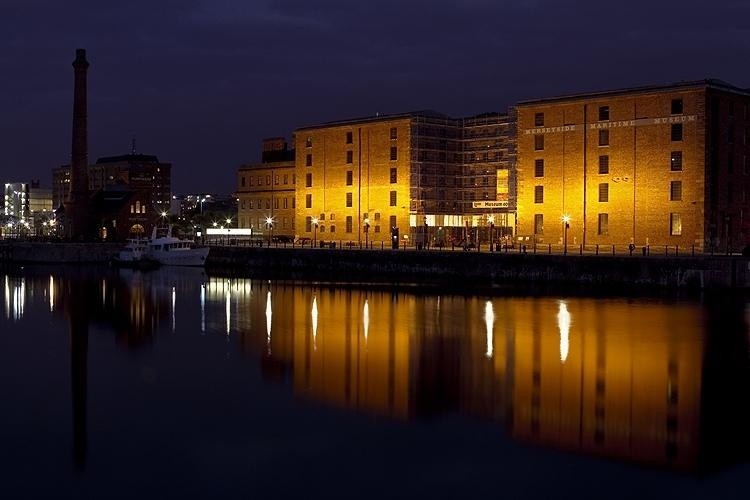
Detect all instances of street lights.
[226,218,232,245]
[266,217,272,246]
[438,225,443,251]
[221,226,225,246]
[563,216,568,252]
[422,216,427,251]
[488,217,494,251]
[313,218,319,247]
[364,218,370,249]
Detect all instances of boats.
[117,224,214,268]
